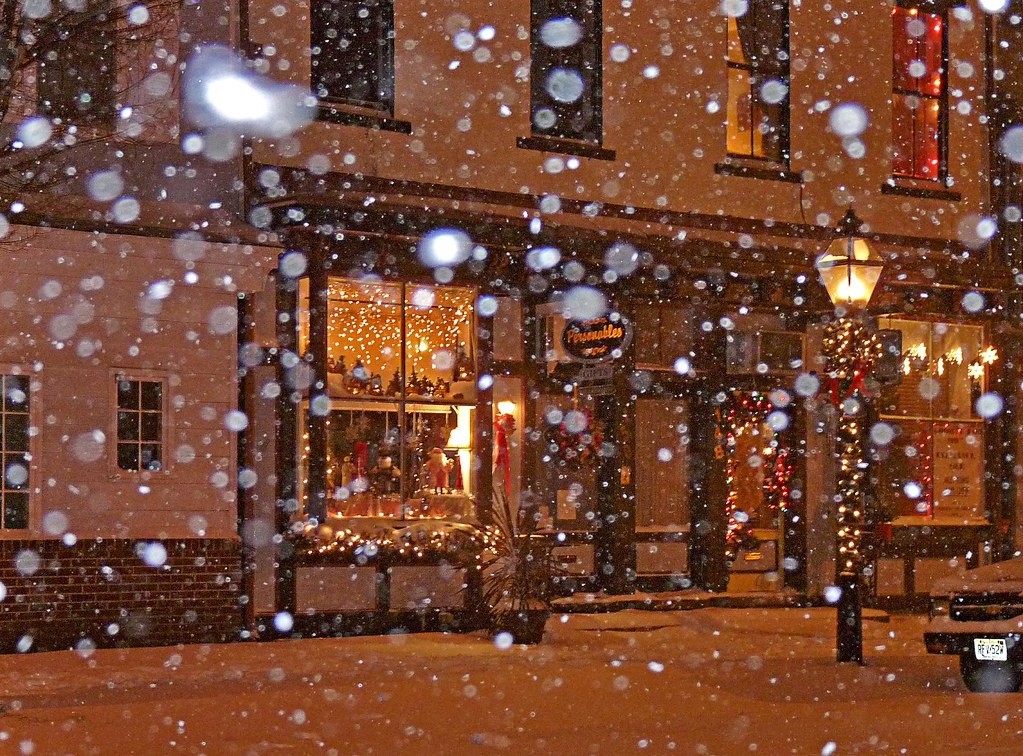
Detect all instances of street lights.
[815,206,883,666]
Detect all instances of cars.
[923,552,1023,694]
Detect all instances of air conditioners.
[726,327,807,377]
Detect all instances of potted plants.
[445,481,580,646]
